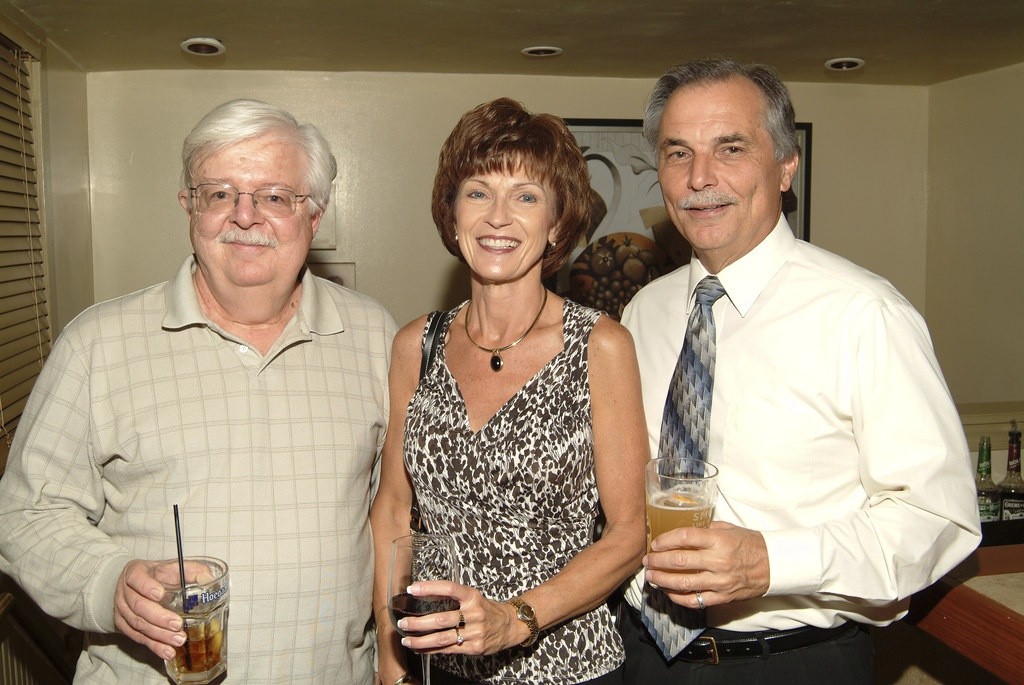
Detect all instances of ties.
[639,278,723,661]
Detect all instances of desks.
[869,517,1024,685]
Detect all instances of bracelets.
[392,671,416,685]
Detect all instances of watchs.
[506,596,540,647]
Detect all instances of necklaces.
[466,284,548,371]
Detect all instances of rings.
[696,591,706,609]
[454,627,464,645]
[455,609,466,628]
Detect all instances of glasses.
[188,181,313,221]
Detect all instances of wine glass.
[387,533,459,685]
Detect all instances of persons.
[615,58,982,685]
[370,98,652,685]
[0,99,397,685]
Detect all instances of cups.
[148,557,227,685]
[645,457,719,594]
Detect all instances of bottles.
[971,436,1002,524]
[998,431,1024,522]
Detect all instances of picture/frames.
[540,117,813,322]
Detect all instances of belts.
[608,602,872,667]
